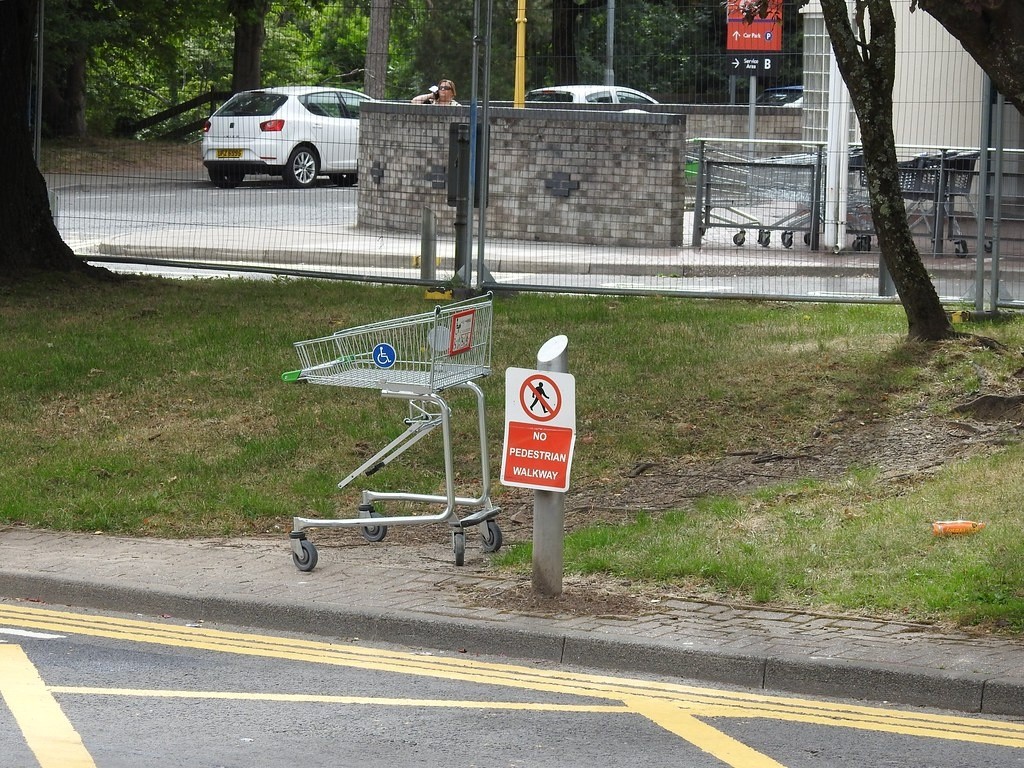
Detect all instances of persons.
[410,79,462,106]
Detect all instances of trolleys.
[280,290,503,572]
[683,138,993,259]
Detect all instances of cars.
[756,85,804,108]
[202,86,376,189]
[525,85,659,104]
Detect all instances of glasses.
[439,86,451,90]
